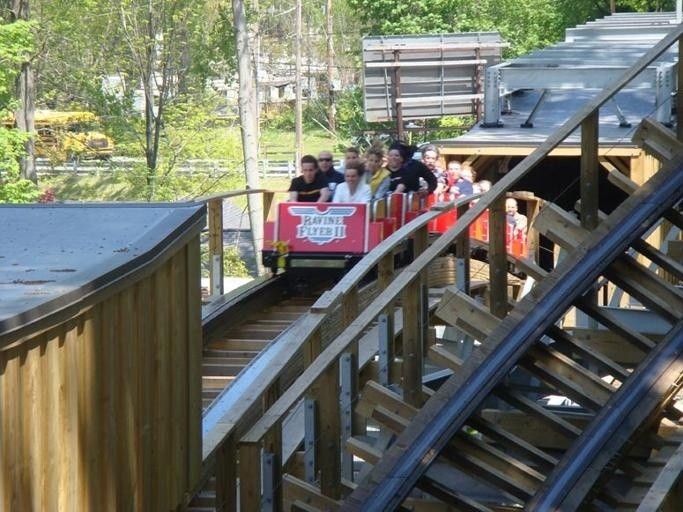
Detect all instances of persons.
[288,138,528,239]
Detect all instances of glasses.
[345,156,357,160]
[318,157,332,163]
